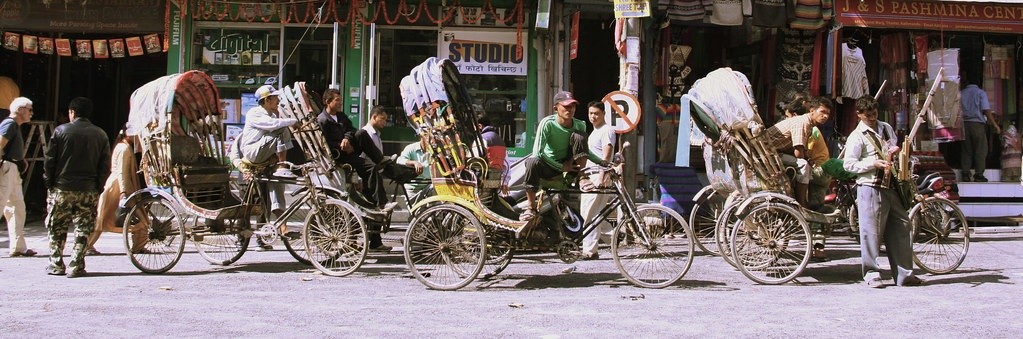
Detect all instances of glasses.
[20,107,33,113]
[806,101,812,103]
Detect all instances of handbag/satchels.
[113,196,140,227]
[895,172,915,211]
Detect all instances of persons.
[754,96,835,262]
[396,140,441,246]
[84,123,150,256]
[959,74,1001,182]
[579,100,626,260]
[230,131,273,252]
[842,95,929,288]
[43,97,112,279]
[348,105,394,252]
[239,85,301,239]
[0,97,38,257]
[1002,125,1023,182]
[468,113,512,260]
[519,90,609,221]
[315,88,397,212]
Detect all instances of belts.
[585,171,599,176]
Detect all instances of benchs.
[460,156,503,207]
[171,136,230,210]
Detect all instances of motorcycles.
[822,174,952,243]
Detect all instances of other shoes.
[865,272,885,289]
[255,243,273,251]
[575,252,600,260]
[902,276,928,286]
[10,248,38,257]
[132,248,151,254]
[377,152,397,170]
[368,240,393,251]
[376,200,398,213]
[960,170,971,182]
[85,248,101,255]
[235,239,247,251]
[273,168,298,178]
[974,174,988,182]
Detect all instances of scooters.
[483,159,586,236]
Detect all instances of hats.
[253,84,282,103]
[552,89,580,106]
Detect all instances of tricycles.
[399,56,694,290]
[122,68,368,277]
[681,68,970,286]
[281,133,516,266]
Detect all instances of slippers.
[813,252,831,261]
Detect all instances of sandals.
[518,204,538,221]
[578,175,595,193]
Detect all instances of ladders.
[23,120,55,193]
